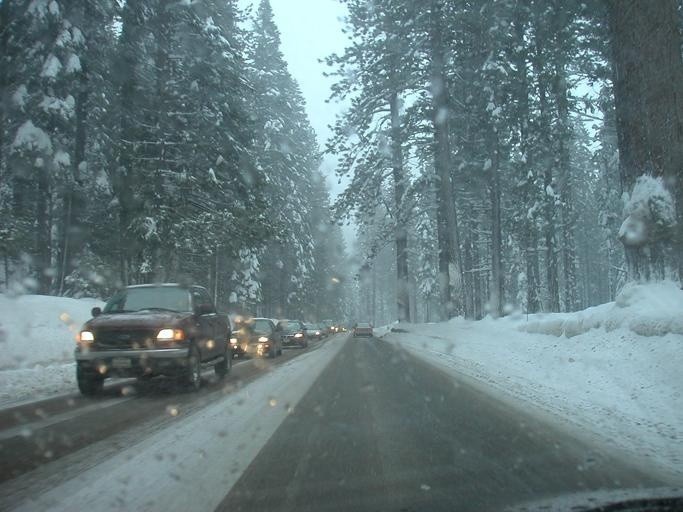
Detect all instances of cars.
[303,318,345,340]
[274,320,307,348]
[352,321,373,338]
[229,318,283,359]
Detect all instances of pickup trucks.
[73,282,233,391]
